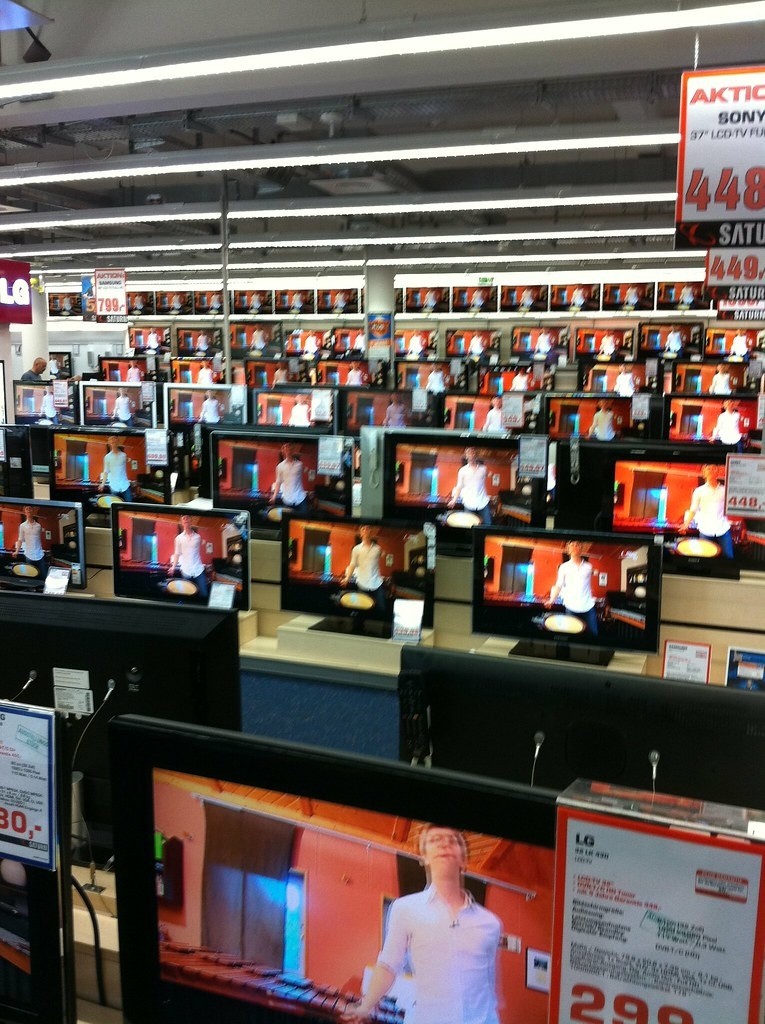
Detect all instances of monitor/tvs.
[471,524,662,652]
[399,645,765,811]
[0,591,243,875]
[0,282,764,573]
[110,713,765,1023]
[110,502,250,611]
[280,510,435,629]
[0,496,88,592]
[0,700,76,1024]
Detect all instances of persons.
[544,539,601,645]
[54,282,762,452]
[166,514,208,606]
[677,463,735,560]
[38,386,58,426]
[267,442,308,521]
[11,505,48,581]
[335,822,504,1024]
[20,357,46,381]
[338,525,387,620]
[444,446,493,526]
[48,355,59,380]
[96,436,131,502]
